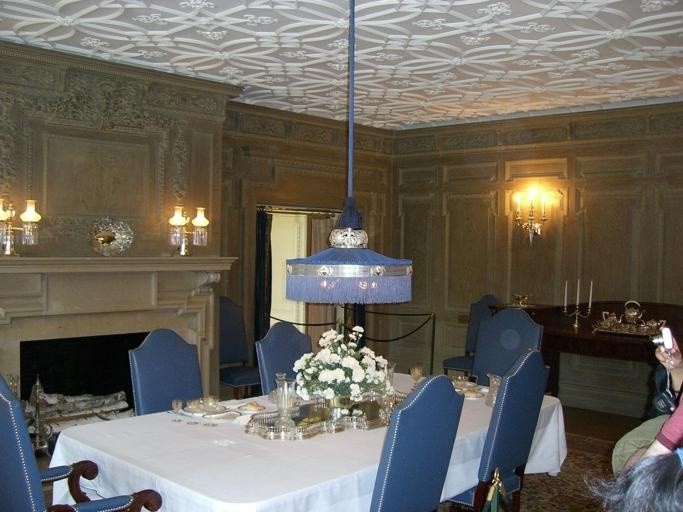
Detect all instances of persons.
[602,335,683,507]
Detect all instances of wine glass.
[271,377,297,429]
[409,367,422,391]
[171,400,183,423]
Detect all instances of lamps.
[0,198,43,255]
[285,0,413,308]
[169,204,210,257]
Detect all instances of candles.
[515,194,547,219]
[563,279,595,311]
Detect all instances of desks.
[540,320,670,419]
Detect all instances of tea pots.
[600,299,660,331]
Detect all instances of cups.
[484,373,501,408]
[376,361,396,409]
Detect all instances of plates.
[450,380,483,400]
[178,403,265,424]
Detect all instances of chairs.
[1,375,161,511]
[218,296,265,398]
[442,295,501,376]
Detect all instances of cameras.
[651,327,673,350]
